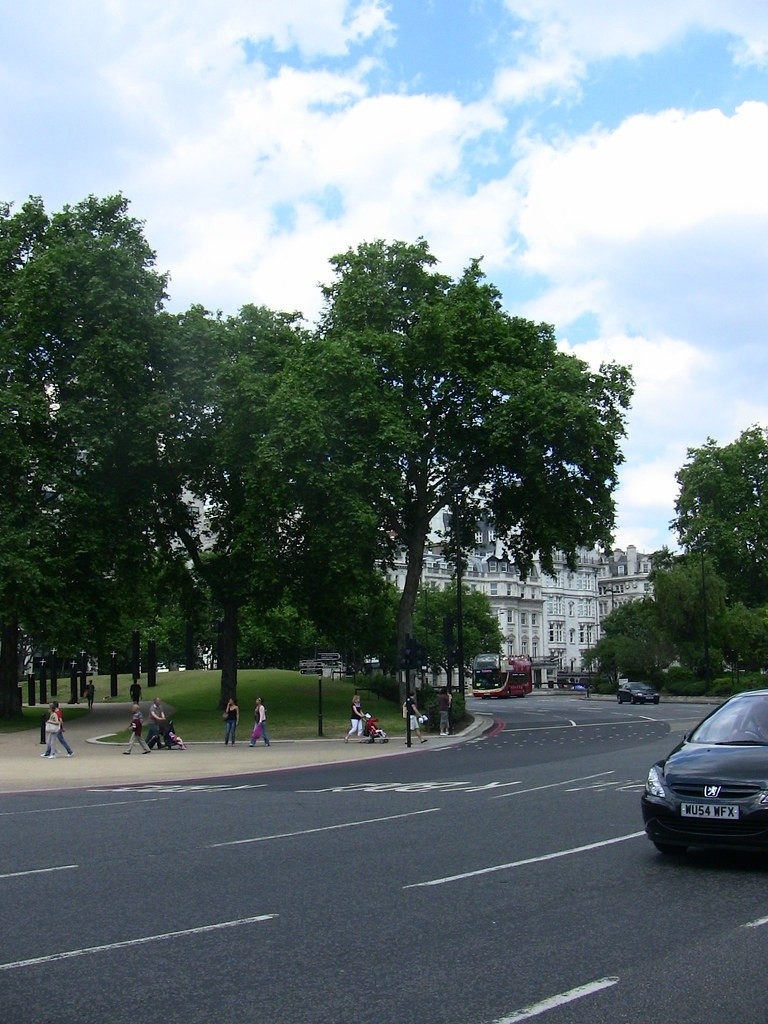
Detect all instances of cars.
[618,682,660,705]
[299,653,346,678]
[642,688,768,861]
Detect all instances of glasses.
[47,708,50,709]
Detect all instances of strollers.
[159,720,186,750]
[362,715,389,744]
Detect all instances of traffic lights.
[402,647,411,668]
[415,649,423,668]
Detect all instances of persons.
[122,704,151,755]
[436,689,452,736]
[129,680,143,705]
[41,700,74,759]
[248,695,271,747]
[85,680,95,710]
[370,720,386,738]
[223,697,239,747]
[345,695,366,743]
[404,690,427,744]
[144,698,187,750]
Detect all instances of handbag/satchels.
[46,713,61,733]
[83,689,89,699]
[223,711,229,721]
[250,725,264,739]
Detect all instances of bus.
[471,653,533,699]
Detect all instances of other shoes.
[440,732,447,736]
[40,752,51,757]
[446,732,450,735]
[47,754,57,759]
[122,752,131,755]
[421,739,428,743]
[65,752,75,757]
[344,737,348,744]
[142,751,151,754]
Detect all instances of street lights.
[691,542,714,696]
